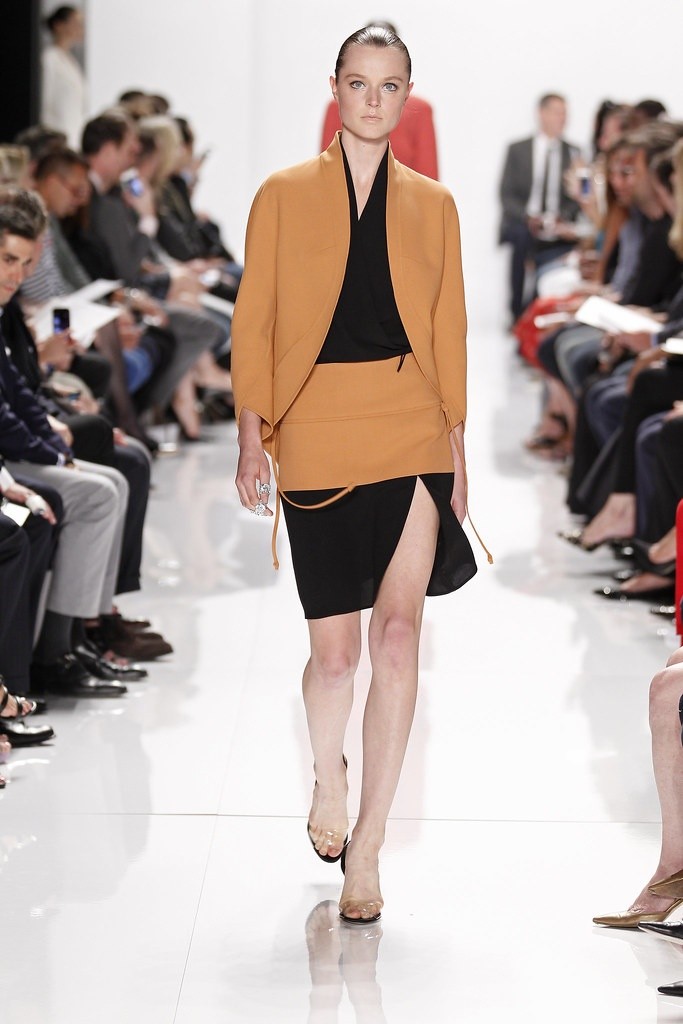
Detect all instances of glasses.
[57,173,84,199]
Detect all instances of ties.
[542,148,552,212]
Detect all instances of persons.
[0,0,244,789]
[231,20,479,926]
[495,92,683,997]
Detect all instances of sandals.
[0,677,37,719]
[307,753,350,863]
[339,841,384,924]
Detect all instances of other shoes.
[0,775,6,788]
[0,734,12,764]
[90,605,172,658]
[524,411,575,462]
[115,419,159,457]
[139,410,177,453]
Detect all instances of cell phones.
[119,171,145,196]
[577,168,591,202]
[53,308,70,334]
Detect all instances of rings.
[259,483,270,495]
[249,502,265,516]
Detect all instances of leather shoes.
[72,639,147,681]
[9,686,47,715]
[648,870,683,900]
[638,921,683,945]
[593,900,682,928]
[28,655,126,696]
[658,981,683,996]
[557,526,676,619]
[0,719,54,747]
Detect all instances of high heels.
[195,380,236,423]
[161,403,214,443]
